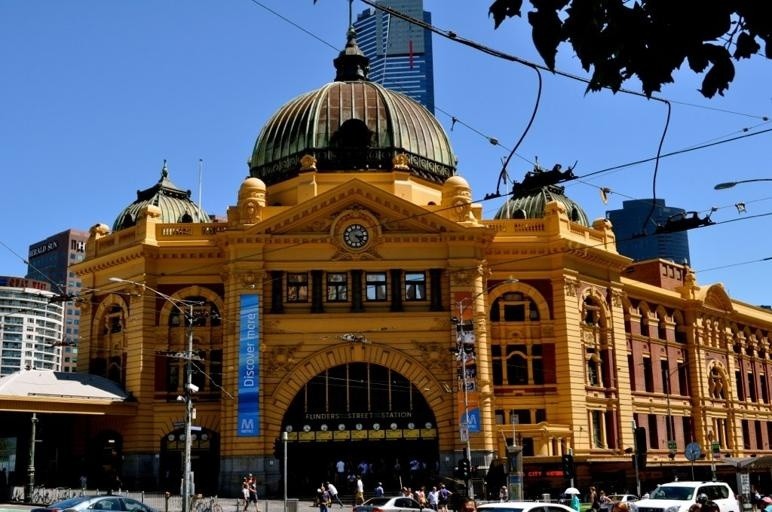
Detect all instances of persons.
[499,485,509,502]
[750,485,772,512]
[79,473,87,492]
[688,493,721,512]
[570,494,581,512]
[241,473,261,512]
[315,458,477,512]
[590,486,630,512]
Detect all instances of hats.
[763,497,772,506]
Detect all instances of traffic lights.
[458,459,470,479]
[561,453,574,479]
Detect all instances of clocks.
[343,223,369,248]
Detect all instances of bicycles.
[196,500,223,512]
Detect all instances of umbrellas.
[564,487,581,494]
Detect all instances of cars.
[30,494,161,512]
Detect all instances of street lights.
[713,178,772,190]
[107,277,218,512]
[281,432,288,512]
[449,278,519,502]
[666,357,713,446]
